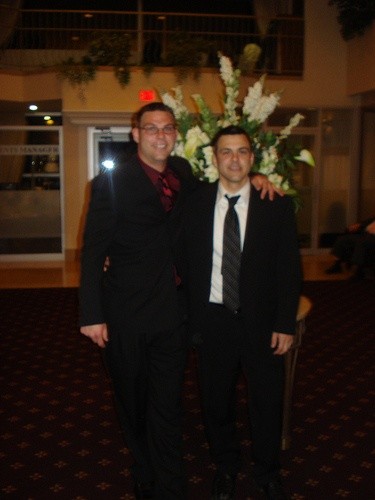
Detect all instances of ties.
[224,195,242,312]
[160,177,176,212]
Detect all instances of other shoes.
[326,262,342,274]
[350,270,364,282]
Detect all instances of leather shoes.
[134,476,189,500]
[257,479,288,500]
[211,472,237,500]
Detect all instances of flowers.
[160,55,316,196]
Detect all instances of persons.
[103,125,302,500]
[78,103,285,500]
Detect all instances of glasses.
[138,126,175,135]
